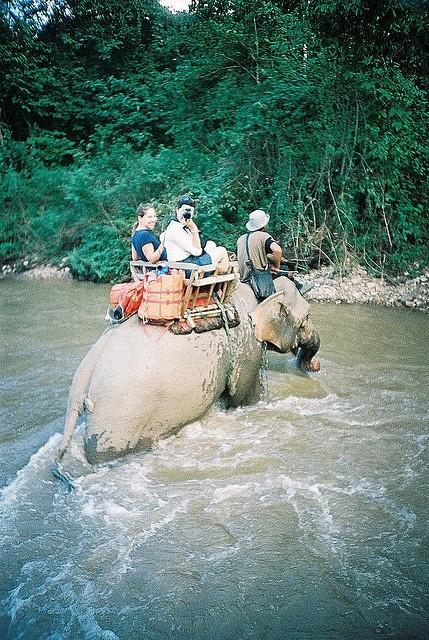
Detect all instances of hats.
[176,196,195,210]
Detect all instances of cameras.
[183,211,191,220]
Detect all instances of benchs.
[129,260,240,313]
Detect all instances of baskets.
[138,270,184,322]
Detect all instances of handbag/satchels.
[245,230,275,301]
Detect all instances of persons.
[130,201,167,268]
[159,194,229,277]
[234,209,282,296]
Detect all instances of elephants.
[50,275,320,492]
